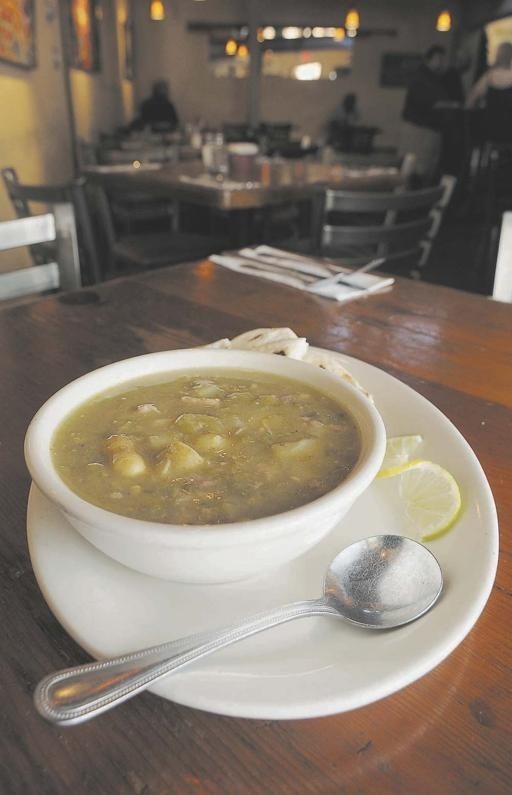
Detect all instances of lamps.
[436,0,451,31]
[150,0,165,22]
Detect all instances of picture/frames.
[0,0,134,82]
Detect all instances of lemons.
[373,434,426,480]
[399,460,461,542]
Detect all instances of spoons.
[238,262,344,288]
[32,534,446,728]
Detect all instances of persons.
[140,79,178,129]
[397,45,462,172]
[464,40,511,148]
[325,90,364,145]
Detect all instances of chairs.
[0,122,457,305]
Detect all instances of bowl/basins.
[23,347,390,589]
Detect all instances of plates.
[26,347,503,723]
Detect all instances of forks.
[257,252,388,279]
